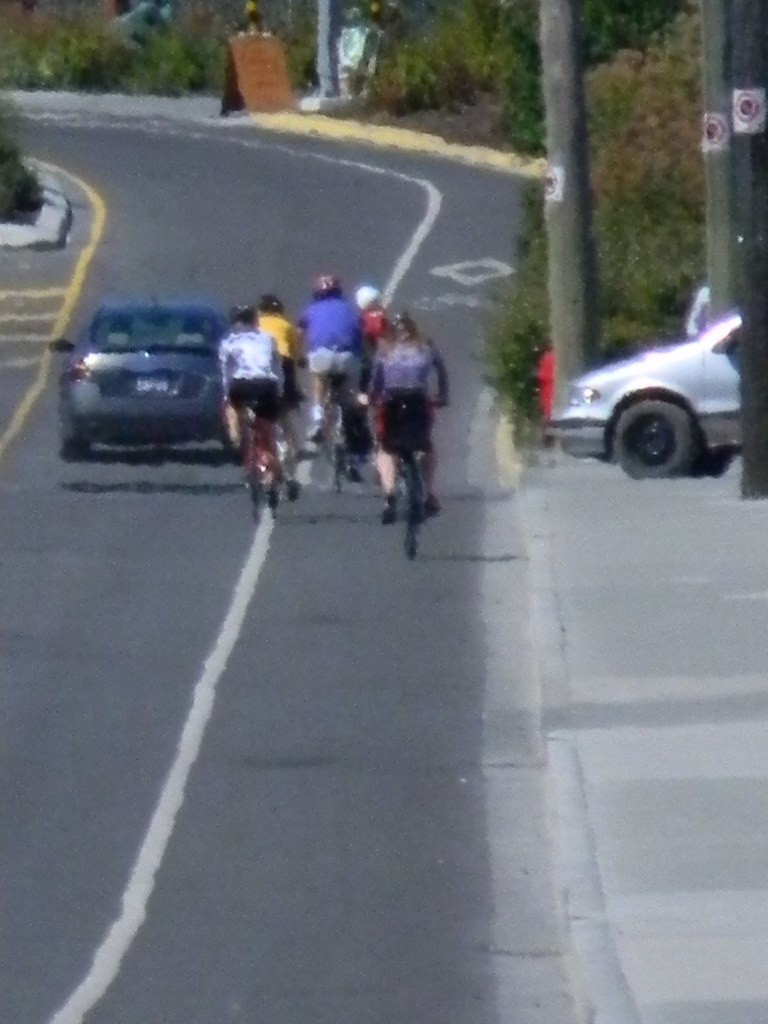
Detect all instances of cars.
[550,307,743,481]
[47,296,243,462]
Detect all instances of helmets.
[259,294,282,311]
[313,275,342,298]
[231,305,254,321]
[356,286,382,311]
[392,319,416,337]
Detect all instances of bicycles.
[245,374,431,560]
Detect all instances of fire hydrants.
[540,350,553,418]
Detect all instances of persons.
[219,295,307,512]
[296,275,388,482]
[359,313,449,526]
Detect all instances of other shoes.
[308,425,320,441]
[382,493,396,524]
[423,494,440,512]
[349,467,362,484]
